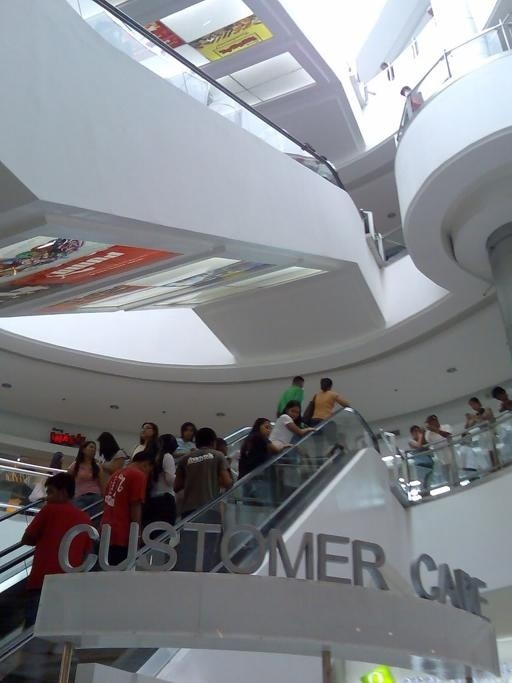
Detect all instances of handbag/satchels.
[301,399,315,424]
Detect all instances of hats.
[425,415,439,423]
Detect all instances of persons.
[294,143,339,182]
[400,87,424,112]
[380,63,395,81]
[407,385,511,498]
